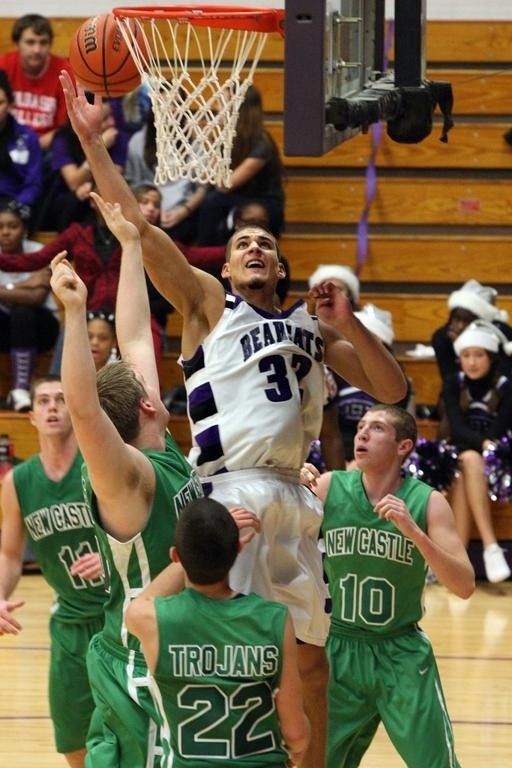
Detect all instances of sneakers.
[5,387,33,414]
[481,543,511,584]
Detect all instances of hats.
[405,344,436,359]
[161,383,189,417]
[309,262,362,311]
[453,317,512,357]
[352,302,396,347]
[448,278,509,324]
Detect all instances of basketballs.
[69,14,149,98]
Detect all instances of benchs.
[1,0,512,567]
